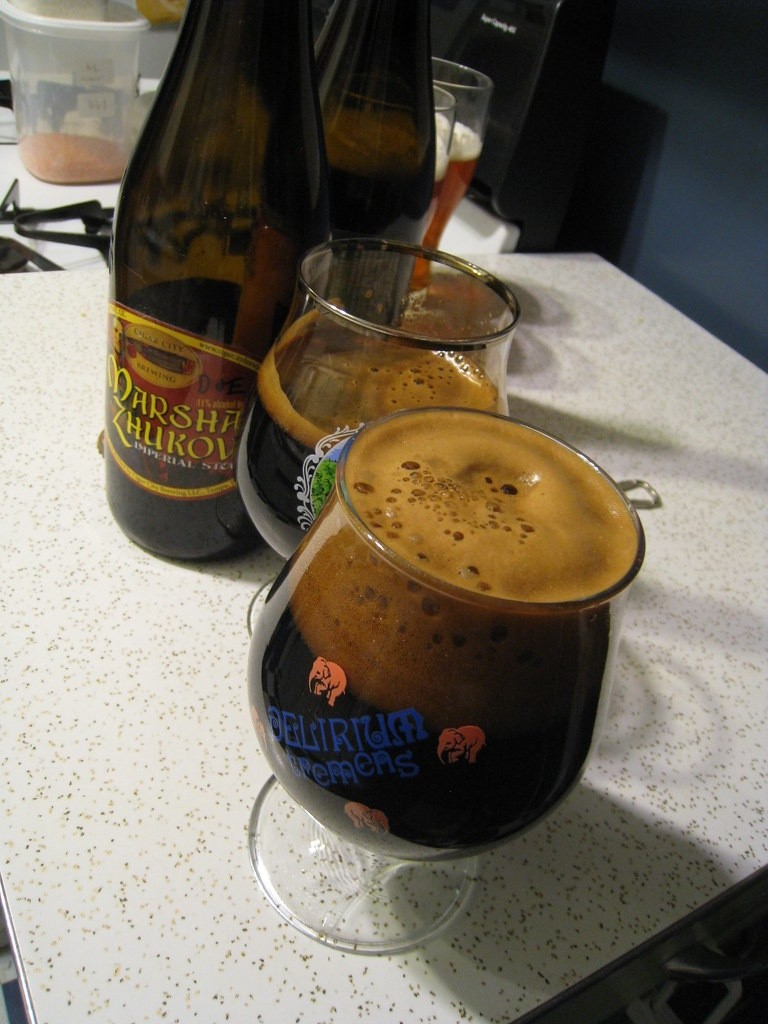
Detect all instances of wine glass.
[244,403,647,955]
[232,238,523,638]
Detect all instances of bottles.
[99,0,332,563]
[309,0,439,333]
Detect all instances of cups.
[400,56,498,319]
[0,0,151,184]
